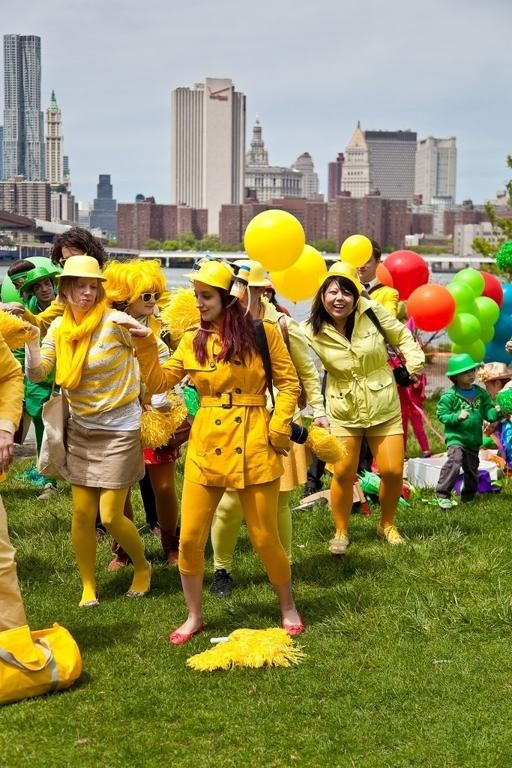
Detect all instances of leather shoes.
[79,521,179,608]
[169,622,204,645]
[283,612,305,636]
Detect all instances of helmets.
[55,255,108,282]
[318,262,364,297]
[445,353,481,377]
[181,258,273,292]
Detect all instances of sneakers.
[435,493,453,509]
[377,519,408,546]
[328,531,350,555]
[37,483,60,501]
[209,568,234,598]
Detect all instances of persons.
[1,225,512,672]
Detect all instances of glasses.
[138,292,161,305]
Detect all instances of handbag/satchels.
[1,622,83,706]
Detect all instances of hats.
[475,362,508,385]
[19,267,62,294]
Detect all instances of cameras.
[269,410,308,445]
[393,368,415,386]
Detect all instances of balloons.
[241,209,511,368]
